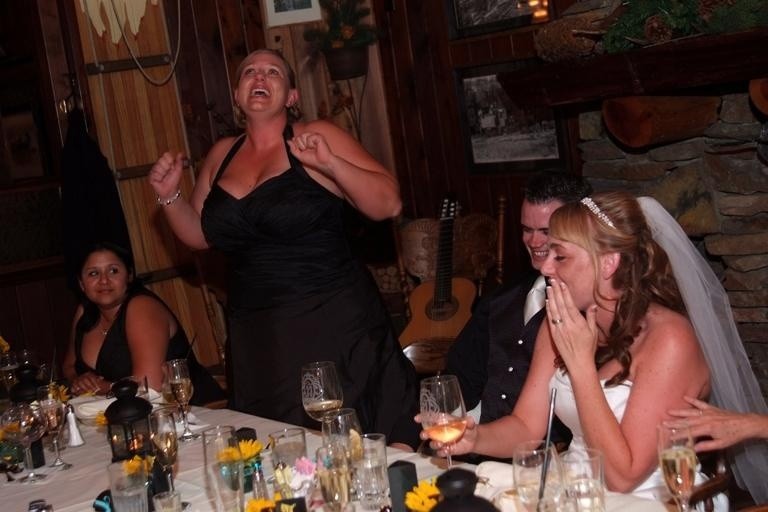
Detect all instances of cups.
[105,459,150,511]
[512,442,561,512]
[268,408,387,511]
[299,358,342,424]
[557,448,605,512]
[152,492,184,512]
[0,350,19,392]
[200,425,246,512]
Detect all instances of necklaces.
[98,324,108,335]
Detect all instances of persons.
[413,189,768,512]
[661,393,767,454]
[387,178,588,464]
[148,47,417,440]
[60,241,227,407]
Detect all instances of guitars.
[398,197,478,372]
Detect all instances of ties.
[523,276,547,327]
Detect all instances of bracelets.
[155,189,181,205]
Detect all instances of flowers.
[303,0,391,51]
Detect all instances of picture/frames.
[454,57,567,176]
[446,0,554,40]
[262,0,324,30]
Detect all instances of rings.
[552,319,562,324]
[699,409,703,414]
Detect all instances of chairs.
[192,253,230,408]
[402,337,457,384]
[392,197,505,318]
[520,424,731,512]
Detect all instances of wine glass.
[149,410,189,510]
[36,385,66,468]
[654,420,698,512]
[418,372,469,469]
[0,404,49,485]
[163,358,200,442]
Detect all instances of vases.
[324,45,369,81]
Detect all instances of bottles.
[250,462,267,503]
[230,425,265,493]
[65,405,85,447]
